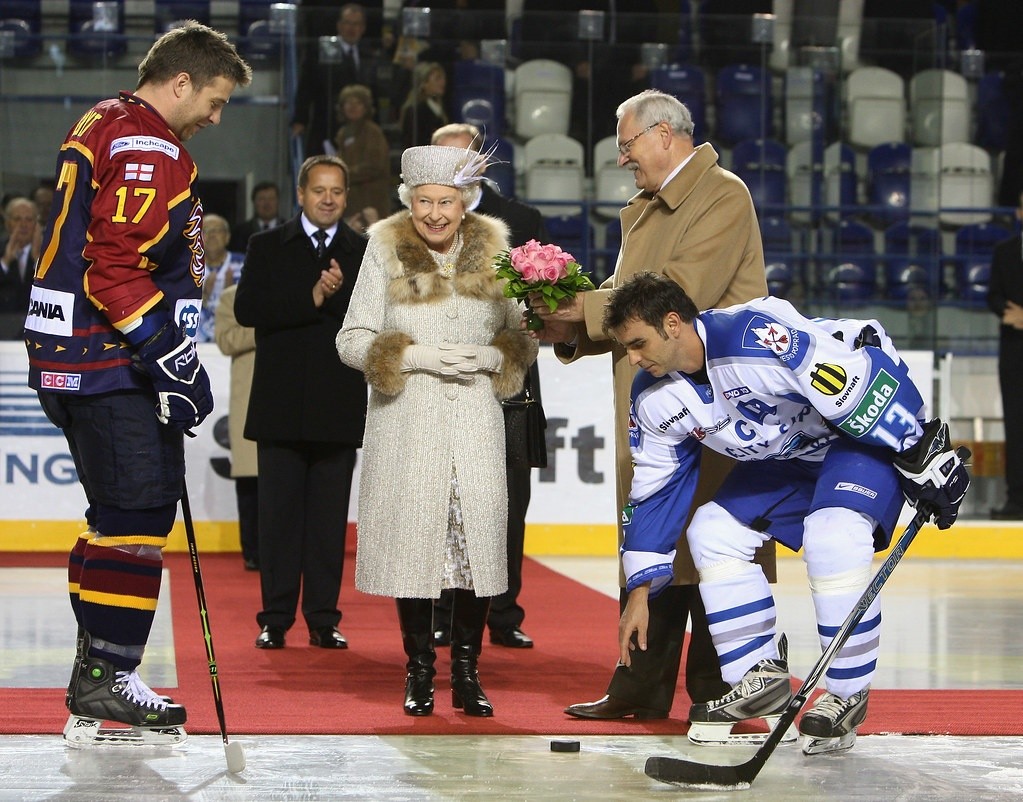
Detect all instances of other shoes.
[991,504,1023,519]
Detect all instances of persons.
[25,20,251,748]
[988,190,1023,521]
[334,144,541,718]
[522,89,772,729]
[0,5,971,755]
[601,270,970,755]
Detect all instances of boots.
[394,597,438,715]
[451,588,493,717]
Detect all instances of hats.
[400,123,510,198]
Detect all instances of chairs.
[0,0,1023,309]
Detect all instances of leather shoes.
[434,628,451,647]
[310,623,349,649]
[256,625,285,649]
[490,624,534,648]
[567,695,671,718]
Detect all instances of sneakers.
[686,630,799,747]
[798,683,871,756]
[63,627,189,749]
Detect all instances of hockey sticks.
[645,442,973,791]
[179,484,247,775]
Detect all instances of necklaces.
[422,231,459,277]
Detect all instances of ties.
[263,223,268,230]
[312,228,330,259]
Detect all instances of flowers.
[491,238,595,330]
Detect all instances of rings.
[332,284,336,289]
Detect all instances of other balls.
[550,740,581,752]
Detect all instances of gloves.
[130,319,214,428]
[892,418,971,531]
[399,343,504,379]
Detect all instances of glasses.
[618,122,660,156]
